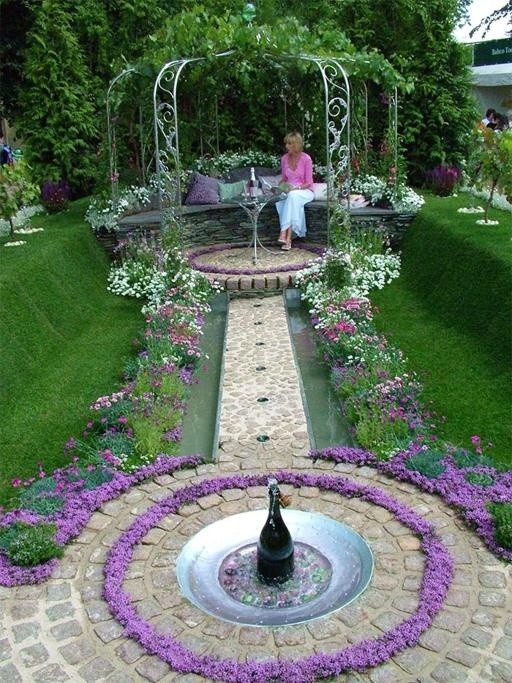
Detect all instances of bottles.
[249,167,257,199]
[258,485,294,586]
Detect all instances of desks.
[226,191,285,263]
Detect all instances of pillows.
[185,166,327,204]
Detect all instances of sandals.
[278,239,291,250]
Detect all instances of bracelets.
[298,186,301,189]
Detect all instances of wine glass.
[242,176,250,201]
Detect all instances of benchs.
[94,165,418,260]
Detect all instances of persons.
[275,132,315,251]
[1,131,11,165]
[480,108,510,132]
